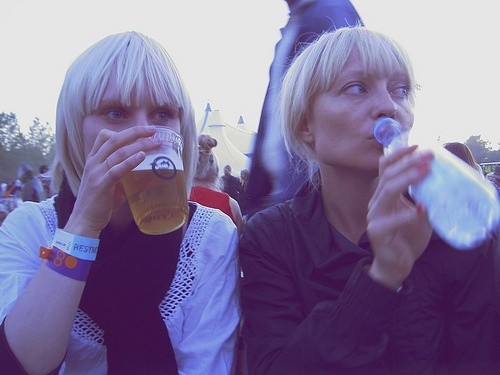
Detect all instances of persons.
[0,31,240,375]
[189,0,500,229]
[0,162,53,225]
[240,16,500,375]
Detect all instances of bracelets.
[52,228,101,260]
[38,246,92,282]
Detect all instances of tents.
[195,102,258,179]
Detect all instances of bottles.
[374,118,500,249]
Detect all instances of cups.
[114,125,187,236]
